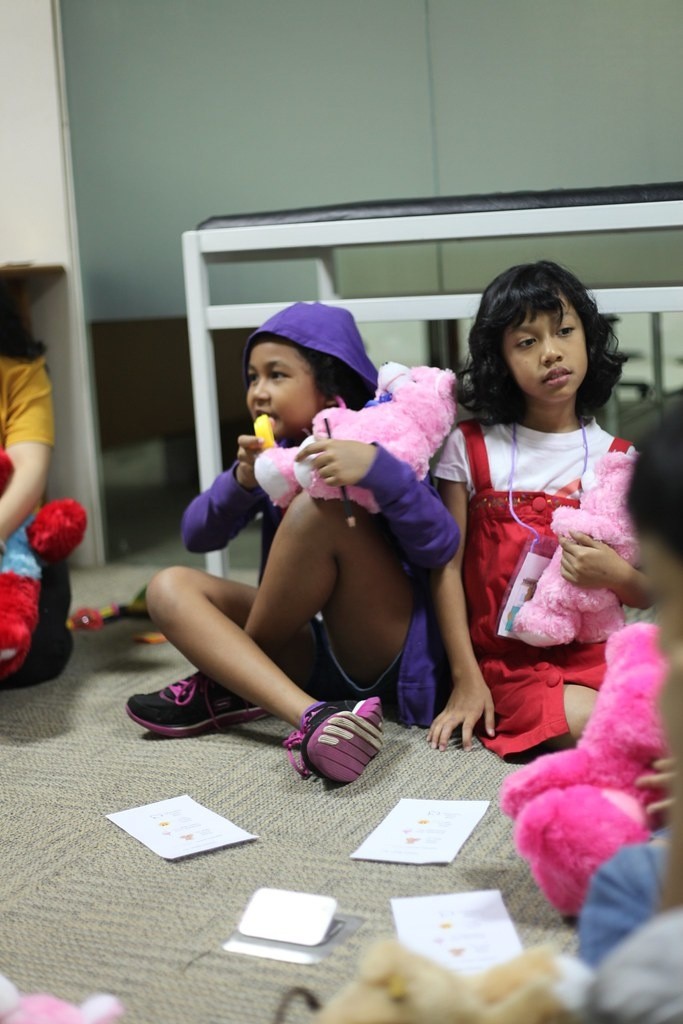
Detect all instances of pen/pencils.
[323,418,356,528]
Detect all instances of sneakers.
[281,694,384,784]
[125,669,267,737]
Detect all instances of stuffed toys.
[0,445,87,679]
[498,622,666,917]
[0,975,124,1024]
[317,943,594,1024]
[254,362,458,513]
[512,450,643,647]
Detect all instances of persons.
[1,274,74,689]
[125,300,461,783]
[576,385,683,1024]
[426,260,657,764]
